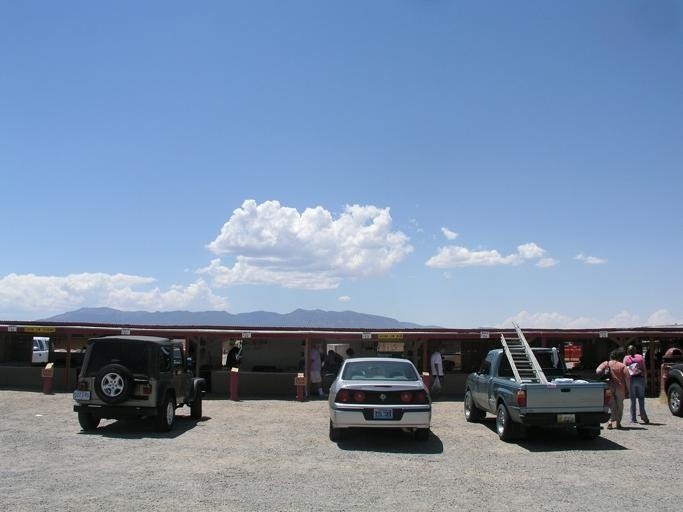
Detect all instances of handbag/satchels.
[600,361,610,382]
[627,357,641,376]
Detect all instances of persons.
[226,347,240,366]
[310,344,329,397]
[618,344,649,423]
[324,350,343,373]
[595,350,631,430]
[427,347,445,392]
[346,347,354,357]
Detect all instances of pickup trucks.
[462,345,612,443]
[438,353,462,370]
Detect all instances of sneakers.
[616,421,621,428]
[631,419,637,423]
[607,421,612,429]
[642,417,649,424]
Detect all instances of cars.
[321,358,431,443]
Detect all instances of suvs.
[32,335,55,364]
[69,336,207,432]
[662,363,683,417]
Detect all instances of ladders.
[500,320,548,383]
